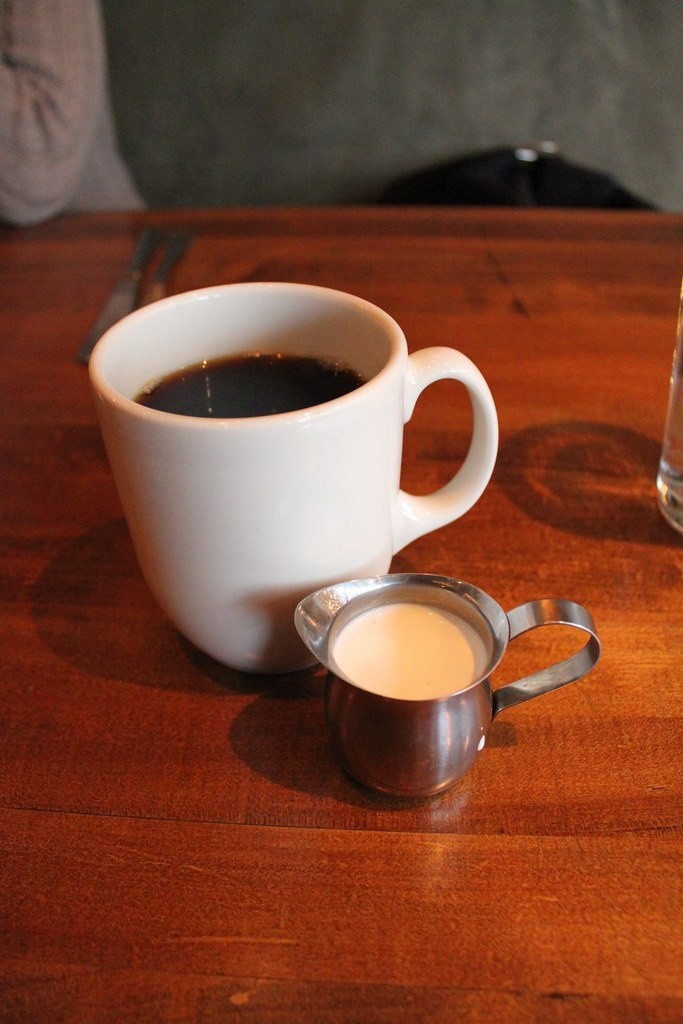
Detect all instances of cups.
[656,277,682,535]
[88,283,499,673]
[294,573,601,799]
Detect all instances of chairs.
[378,149,663,210]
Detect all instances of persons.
[0,1,148,227]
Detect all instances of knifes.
[75,219,161,365]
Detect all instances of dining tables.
[0,206,683,1024]
